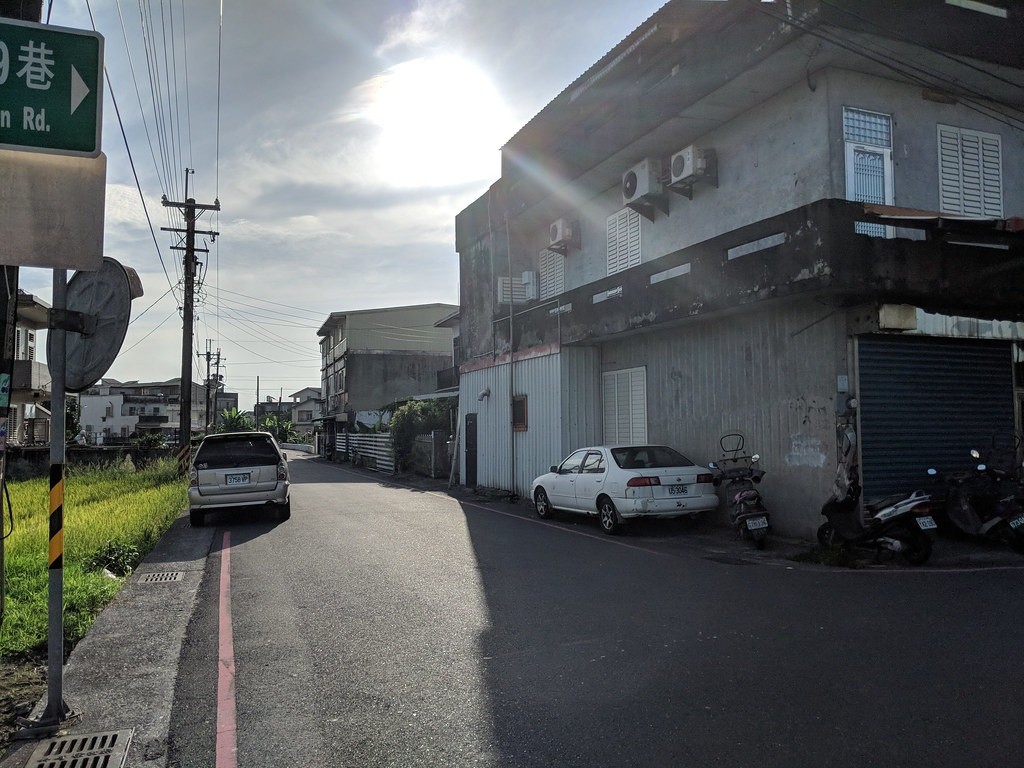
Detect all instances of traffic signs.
[0,17,104,158]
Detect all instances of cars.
[187,431,291,527]
[530,444,720,536]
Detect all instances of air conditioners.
[621,157,664,207]
[549,218,572,248]
[671,144,707,182]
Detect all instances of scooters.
[816,423,938,568]
[707,429,773,550]
[926,427,1023,555]
